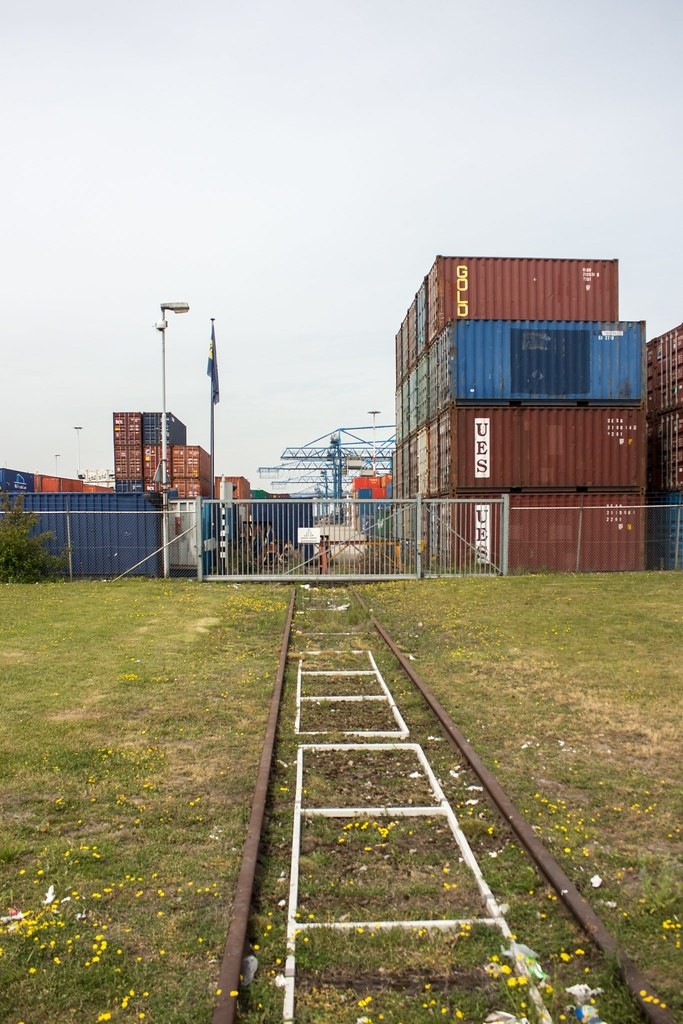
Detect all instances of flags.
[207,324,220,405]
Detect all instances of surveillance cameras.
[156,321,166,331]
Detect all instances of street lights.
[160,301,189,579]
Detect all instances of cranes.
[257,424,396,522]
